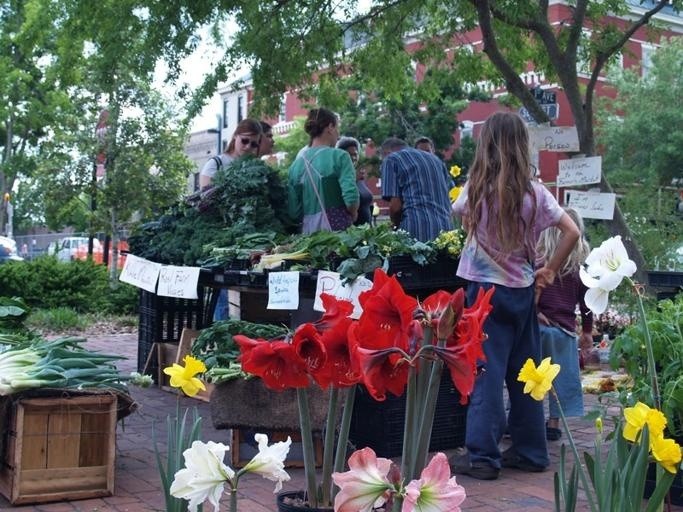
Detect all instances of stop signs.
[92,109,113,166]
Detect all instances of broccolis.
[149,214,222,264]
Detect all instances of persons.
[257,122,274,161]
[380,138,454,245]
[503,207,590,441]
[198,118,262,195]
[289,108,359,271]
[414,136,455,193]
[450,112,580,479]
[335,136,372,228]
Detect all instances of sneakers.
[438,443,499,481]
[496,442,543,473]
[545,420,561,440]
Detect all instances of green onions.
[0,337,154,396]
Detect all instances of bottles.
[599,334,613,361]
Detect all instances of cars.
[53,236,105,266]
[0,235,17,262]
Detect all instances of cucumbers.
[0,333,27,345]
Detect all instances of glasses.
[234,132,260,149]
